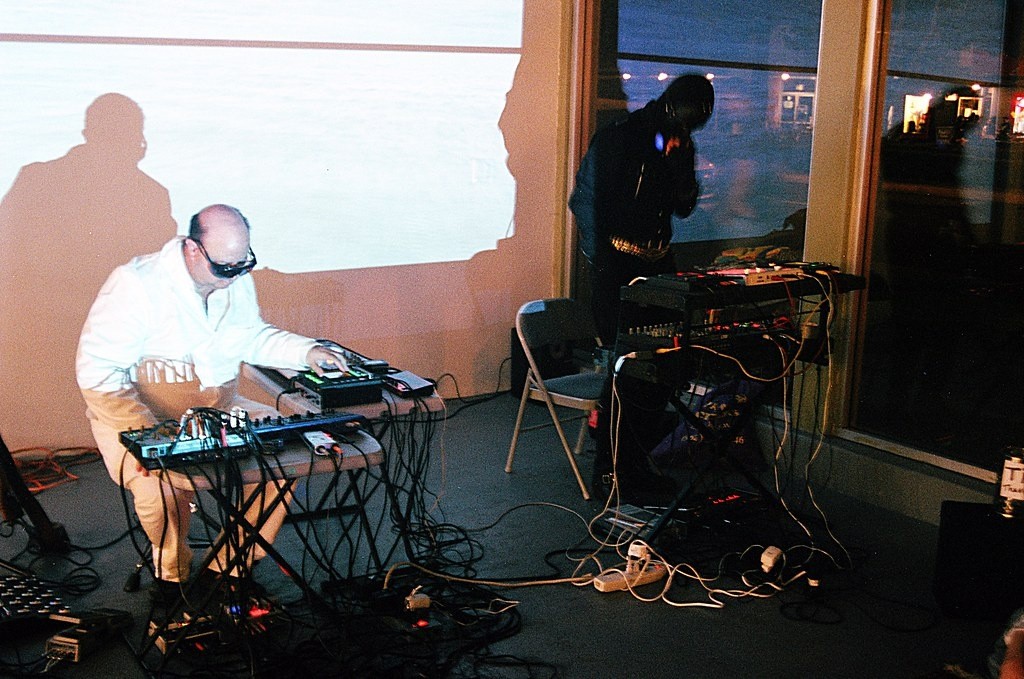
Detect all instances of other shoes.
[207,569,266,599]
[147,576,180,602]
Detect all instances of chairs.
[504,297,607,502]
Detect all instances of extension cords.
[594,564,666,592]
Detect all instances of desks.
[242,364,444,589]
[122,431,384,679]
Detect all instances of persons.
[76,205,349,622]
[569,74,715,351]
[986,607,1024,679]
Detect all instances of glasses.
[193,238,257,278]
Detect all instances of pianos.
[619,261,866,311]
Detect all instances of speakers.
[510,328,572,406]
